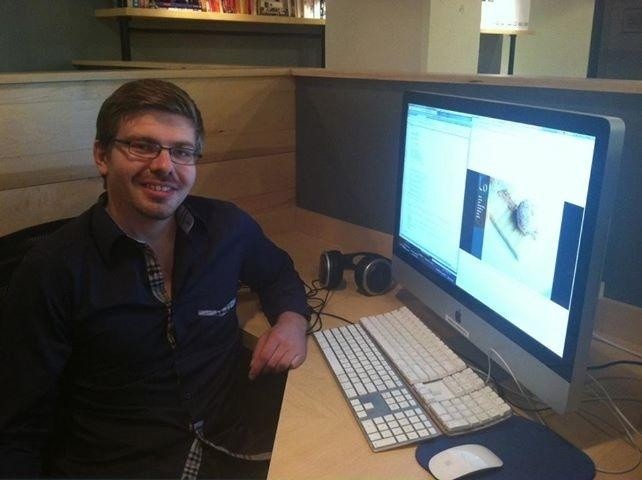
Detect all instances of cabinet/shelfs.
[71,1,326,71]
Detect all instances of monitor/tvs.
[393,88,626,417]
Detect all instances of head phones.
[319,249,392,297]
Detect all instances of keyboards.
[312,322,443,453]
[358,305,515,438]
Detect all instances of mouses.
[428,443,504,476]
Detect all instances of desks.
[238,205,642,480]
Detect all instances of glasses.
[110,138,203,166]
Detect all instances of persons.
[2,78,312,480]
